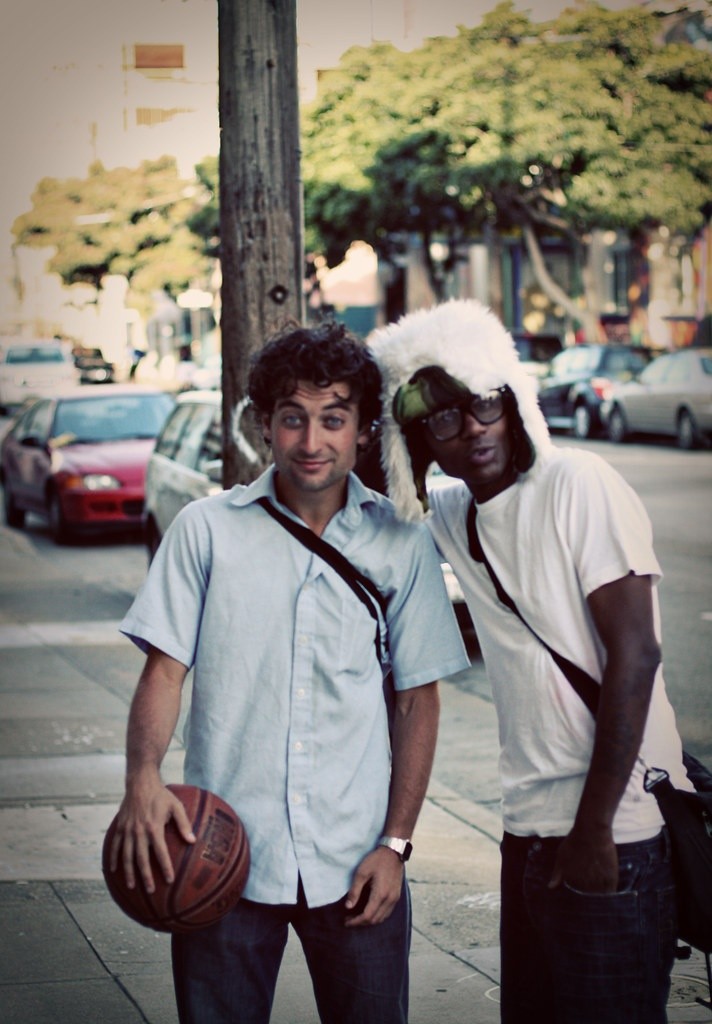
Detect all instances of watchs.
[377,837,413,862]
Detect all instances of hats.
[363,299,551,523]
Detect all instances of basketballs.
[102,783,250,933]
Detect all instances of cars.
[512,335,712,451]
[0,340,224,569]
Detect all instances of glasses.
[417,389,506,442]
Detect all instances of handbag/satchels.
[644,750,712,952]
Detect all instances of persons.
[110,323,472,1024]
[367,300,697,1024]
